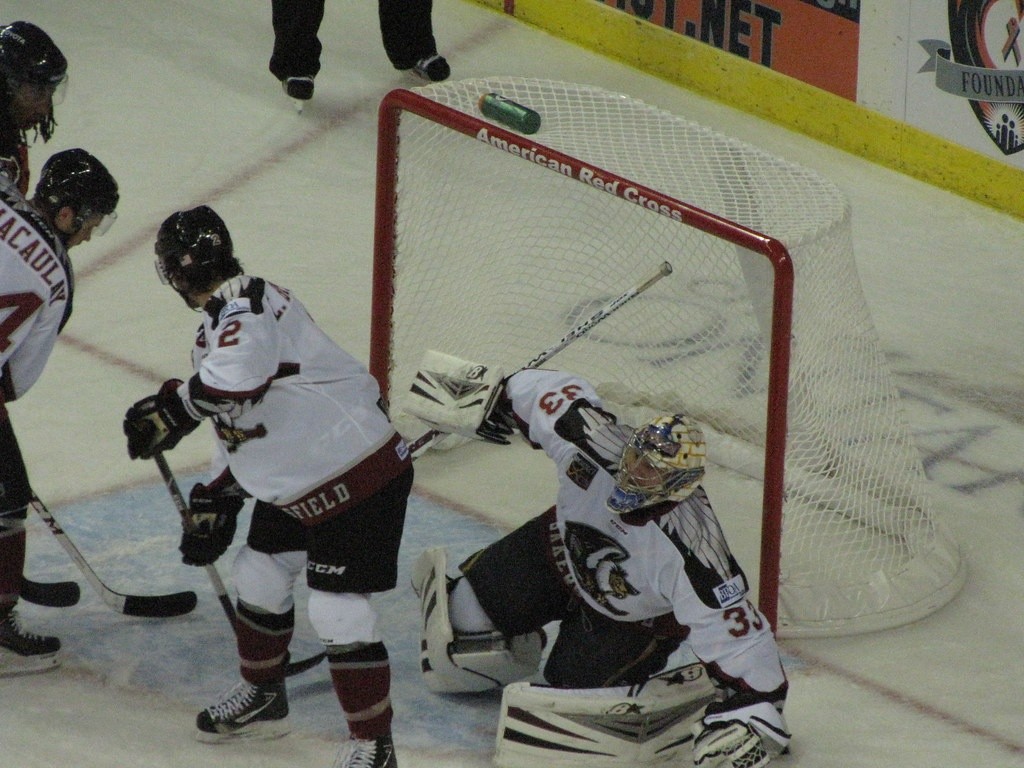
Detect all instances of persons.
[267,0,453,115]
[0,18,68,197]
[121,206,414,768]
[404,349,794,768]
[1,149,123,678]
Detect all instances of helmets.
[0,19,68,86]
[35,147,120,216]
[155,204,233,274]
[604,412,707,516]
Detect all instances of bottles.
[478,93,541,134]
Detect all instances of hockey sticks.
[406,260,675,460]
[28,489,199,618]
[137,414,328,676]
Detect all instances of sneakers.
[0,601,62,675]
[331,731,398,768]
[281,74,316,115]
[412,53,450,81]
[195,651,292,744]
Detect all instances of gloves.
[124,378,200,460]
[178,482,245,567]
